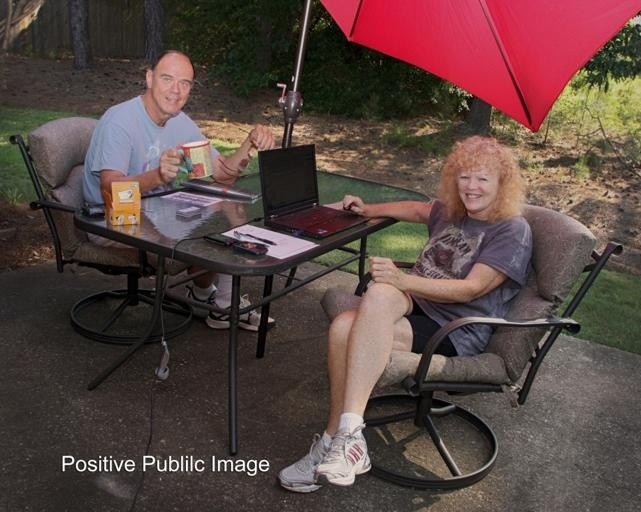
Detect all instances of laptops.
[257,143,371,239]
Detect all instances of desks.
[69,164,433,456]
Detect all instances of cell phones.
[203,231,238,247]
[234,239,268,256]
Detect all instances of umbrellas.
[255,0,641,360]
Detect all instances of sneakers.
[206,293,277,332]
[314,423,372,488]
[185,284,218,315]
[278,432,333,494]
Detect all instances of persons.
[276,133,534,494]
[81,46,278,335]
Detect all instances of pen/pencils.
[246,234,277,245]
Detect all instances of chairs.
[351,199,625,491]
[9,115,198,344]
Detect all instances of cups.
[174,142,213,180]
